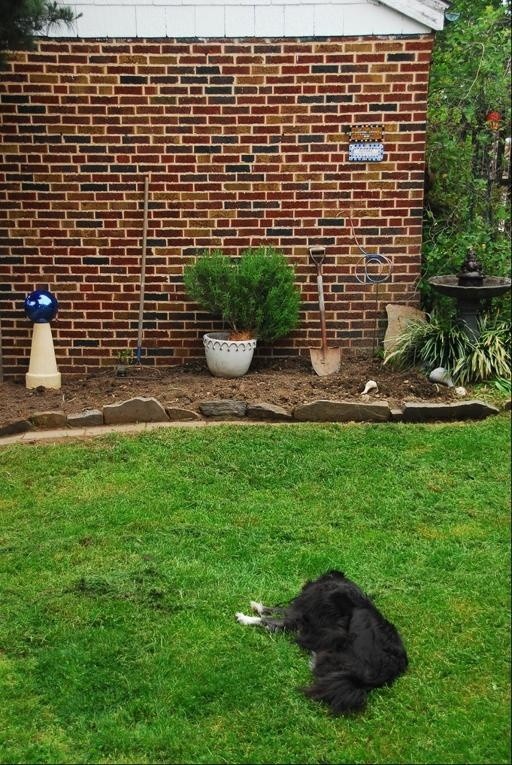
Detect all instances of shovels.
[309,246,341,377]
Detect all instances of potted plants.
[182,244,301,377]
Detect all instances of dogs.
[234,569,409,718]
